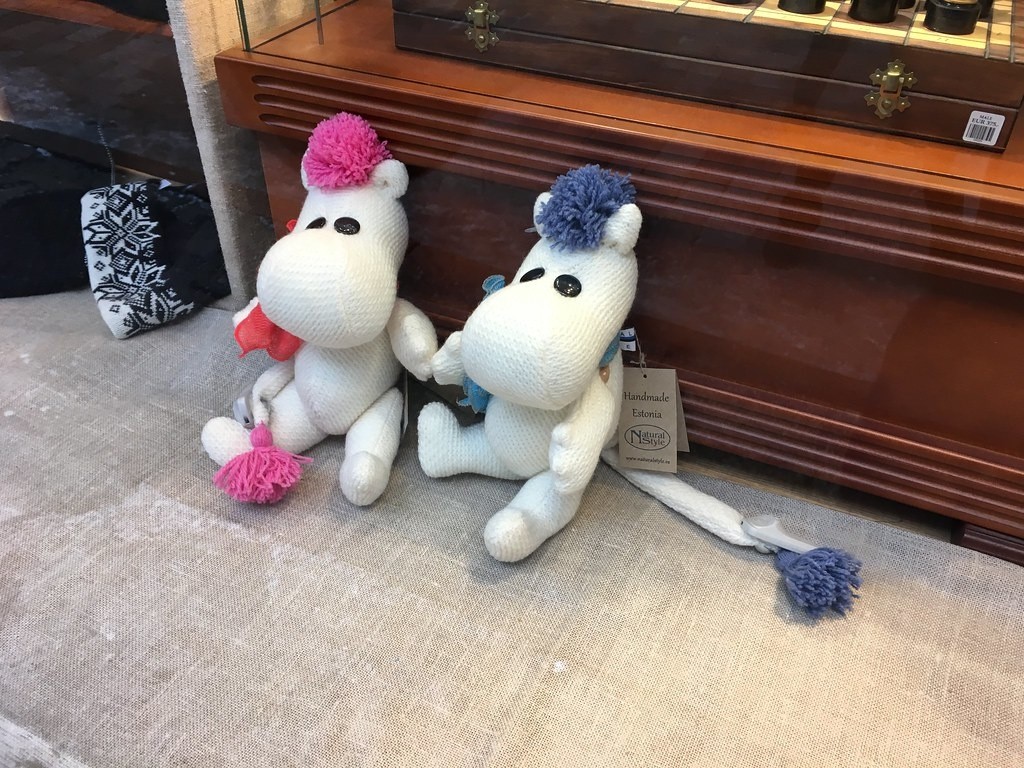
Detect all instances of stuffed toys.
[200,113,439,509]
[418,164,865,623]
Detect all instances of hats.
[80,178,231,340]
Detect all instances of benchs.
[0,7,205,185]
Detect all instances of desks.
[213,0,1024,567]
